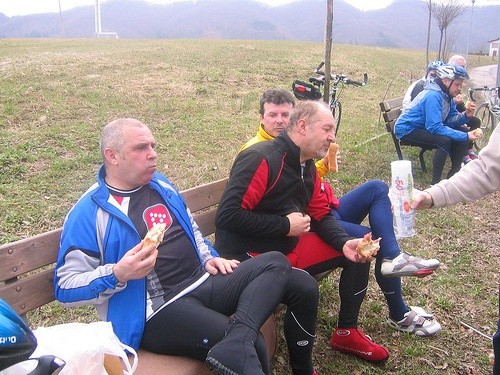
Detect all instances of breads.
[327,143,340,172]
[356,232,382,258]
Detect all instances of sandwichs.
[142,223,166,254]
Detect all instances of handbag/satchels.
[0,321,138,375]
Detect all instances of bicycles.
[470,84,499,153]
[293,61,369,136]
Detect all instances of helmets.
[0,298,38,365]
[436,64,470,79]
[428,61,445,69]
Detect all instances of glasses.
[430,72,436,77]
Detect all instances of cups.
[391,160,415,237]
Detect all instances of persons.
[393,56,500,375]
[213,100,389,375]
[54,119,293,375]
[238,89,442,336]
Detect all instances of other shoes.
[462,155,472,165]
[447,167,461,179]
[205,322,266,375]
[430,178,440,186]
[330,327,389,361]
[468,149,478,160]
[291,368,319,375]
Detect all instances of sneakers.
[387,311,441,336]
[381,251,440,278]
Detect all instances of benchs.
[0,177,338,375]
[379,97,438,173]
